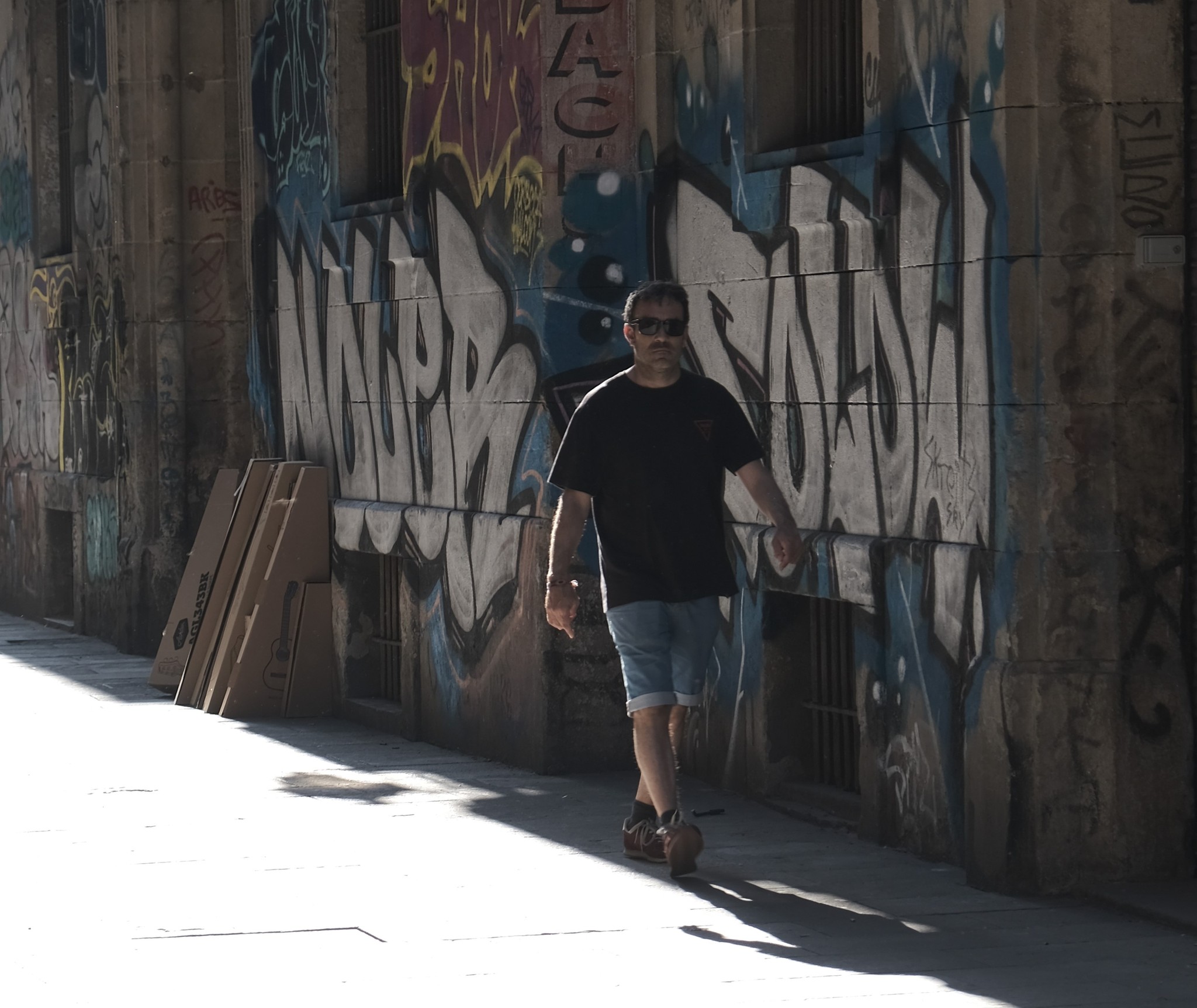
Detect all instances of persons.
[546,281,806,875]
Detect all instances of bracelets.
[544,576,572,588]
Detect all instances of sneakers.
[656,808,704,879]
[622,816,667,863]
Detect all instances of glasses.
[628,318,687,336]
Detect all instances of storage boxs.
[148,457,334,722]
